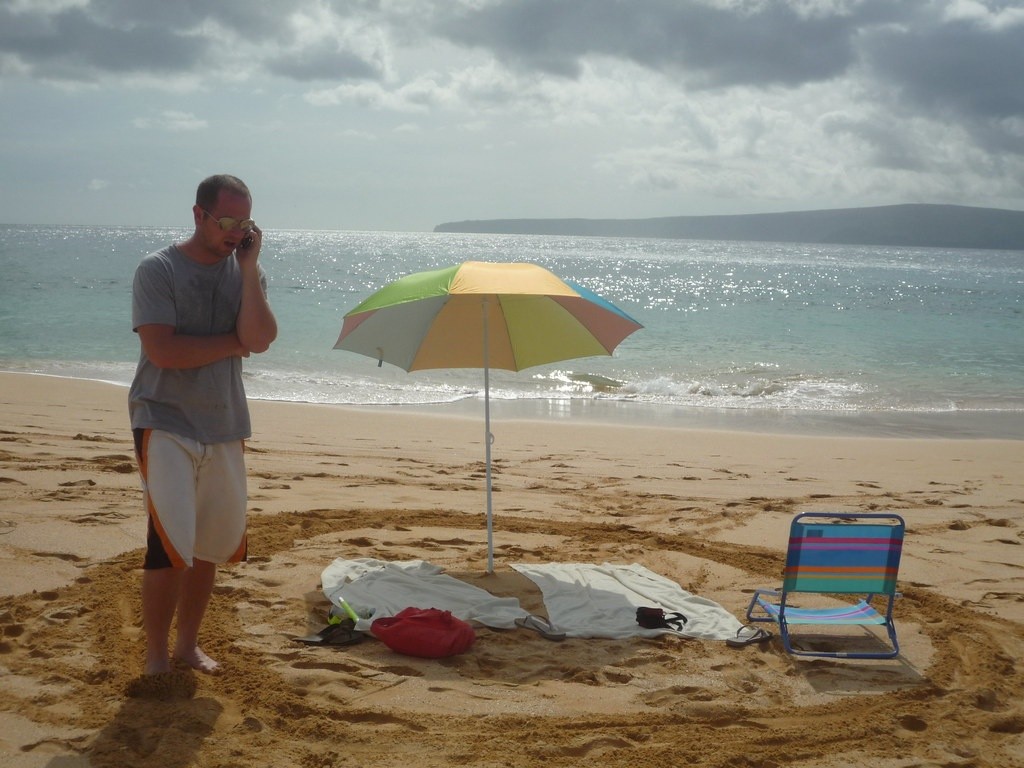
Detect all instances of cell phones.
[240,234,252,249]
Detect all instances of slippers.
[515,615,566,640]
[292,622,364,645]
[725,624,775,647]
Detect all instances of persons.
[128,174,277,675]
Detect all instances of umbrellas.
[332,262,645,571]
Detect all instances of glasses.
[202,207,255,231]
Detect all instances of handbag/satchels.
[636,606,688,631]
[370,607,475,657]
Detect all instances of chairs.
[746,511,905,660]
